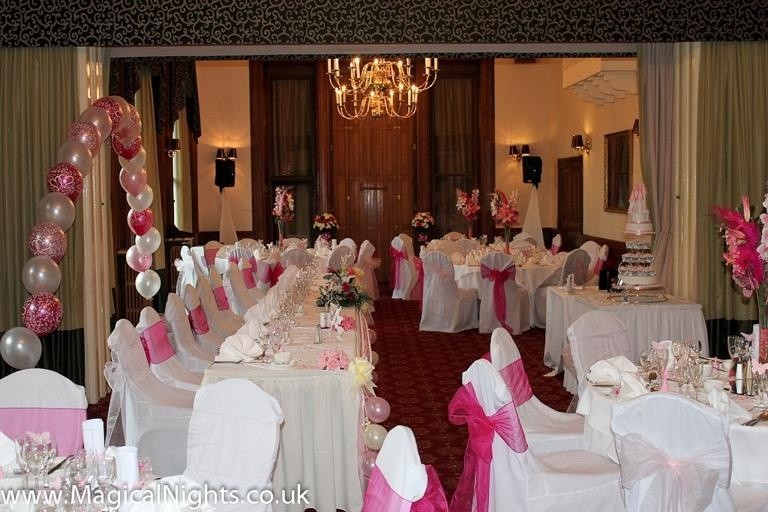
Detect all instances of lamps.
[326,56,440,120]
[166,138,181,158]
[570,134,592,156]
[214,146,238,161]
[510,144,530,161]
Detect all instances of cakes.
[616,183,664,290]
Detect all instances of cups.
[702,363,713,377]
[60,448,118,512]
[16,433,57,492]
[703,379,724,396]
[273,352,291,364]
[638,346,670,391]
[138,458,153,484]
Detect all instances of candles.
[350,59,355,80]
[398,60,402,76]
[426,68,430,75]
[328,59,331,73]
[415,87,417,103]
[408,89,411,105]
[373,58,378,70]
[399,83,402,102]
[353,85,357,102]
[389,91,394,108]
[406,58,410,65]
[335,87,339,104]
[434,57,437,70]
[370,91,375,106]
[425,57,430,67]
[334,59,339,70]
[342,84,346,103]
[338,90,342,106]
[407,66,410,77]
[412,85,415,103]
[356,58,360,78]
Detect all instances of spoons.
[743,411,768,427]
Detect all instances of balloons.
[24,257,59,296]
[116,148,147,170]
[126,245,154,272]
[34,192,75,232]
[371,372,379,384]
[29,222,66,262]
[368,326,376,343]
[127,186,155,212]
[135,228,162,256]
[94,96,121,134]
[366,424,388,451]
[112,96,131,132]
[135,269,160,301]
[119,167,147,198]
[66,121,100,152]
[81,106,113,144]
[20,293,62,336]
[125,208,153,237]
[56,139,92,177]
[113,108,145,158]
[0,326,40,371]
[362,450,377,476]
[364,395,390,424]
[47,164,81,201]
[373,350,380,366]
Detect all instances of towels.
[214,332,264,366]
[216,264,301,338]
[608,353,640,378]
[588,360,628,388]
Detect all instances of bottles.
[735,361,744,394]
[746,361,753,395]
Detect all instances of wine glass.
[259,260,318,343]
[755,373,768,408]
[670,336,703,401]
[254,334,276,364]
[739,336,750,360]
[727,335,740,360]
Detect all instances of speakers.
[214,159,235,187]
[523,156,542,183]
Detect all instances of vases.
[278,218,283,250]
[467,221,472,239]
[755,287,768,368]
[504,227,511,253]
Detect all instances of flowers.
[714,199,768,294]
[272,186,295,221]
[317,266,368,308]
[312,212,339,233]
[456,187,480,221]
[410,212,435,241]
[489,189,520,227]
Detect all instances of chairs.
[140,306,203,387]
[184,285,224,350]
[478,253,530,335]
[461,360,623,512]
[358,426,447,512]
[1,370,86,470]
[167,293,214,365]
[107,321,196,478]
[614,393,737,512]
[223,262,258,315]
[390,233,423,299]
[145,378,281,505]
[565,311,631,385]
[210,270,243,327]
[355,239,380,301]
[490,327,585,442]
[551,234,561,254]
[534,250,590,327]
[584,244,609,286]
[175,247,198,284]
[203,236,356,268]
[419,251,478,333]
[198,277,238,332]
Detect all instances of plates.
[703,373,718,379]
[271,362,294,367]
[700,392,728,396]
